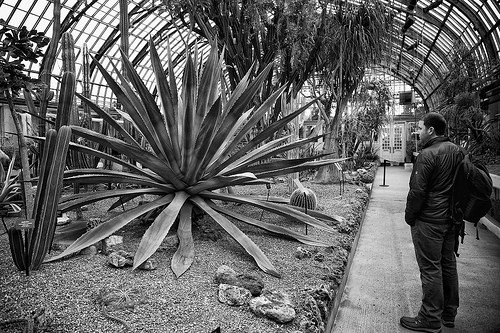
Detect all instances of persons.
[400,112,461,333]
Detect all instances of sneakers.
[400,315,443,333]
[441,319,455,331]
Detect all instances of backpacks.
[449,146,494,224]
